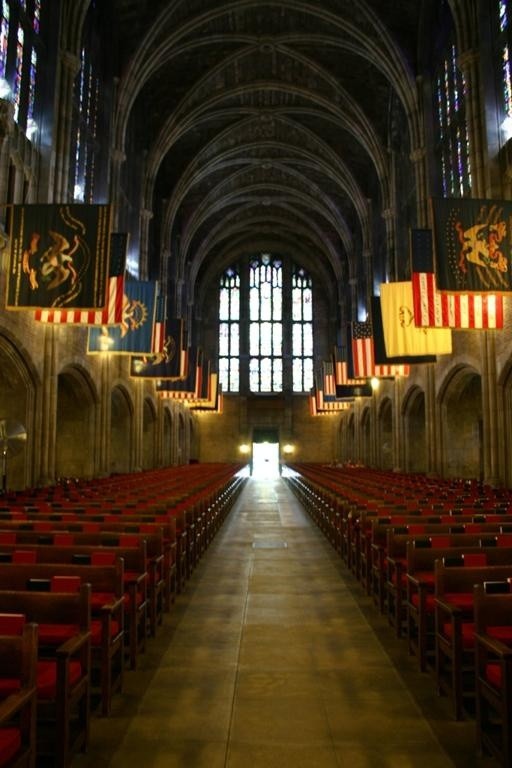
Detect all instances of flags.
[5,202,223,414]
[306,200,512,417]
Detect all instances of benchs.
[0,461,250,768]
[281,462,511,767]
